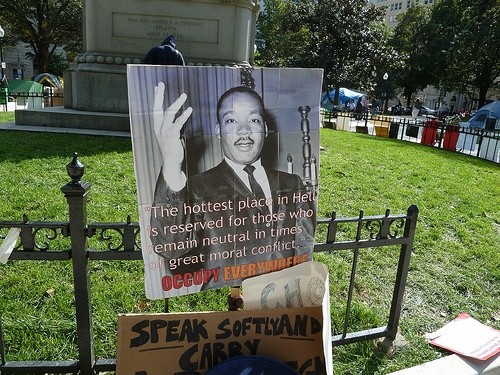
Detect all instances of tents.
[322,86,366,110]
[459,100,500,132]
[0,73,64,106]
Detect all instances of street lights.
[0,25,5,112]
[381,73,388,113]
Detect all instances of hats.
[161,35,176,48]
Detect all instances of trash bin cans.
[443,124,461,151]
[484,117,497,131]
[420,121,439,146]
[0,84,8,104]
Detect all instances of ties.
[243,165,270,216]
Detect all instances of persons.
[149,82,316,290]
[355,95,469,121]
[143,35,185,65]
